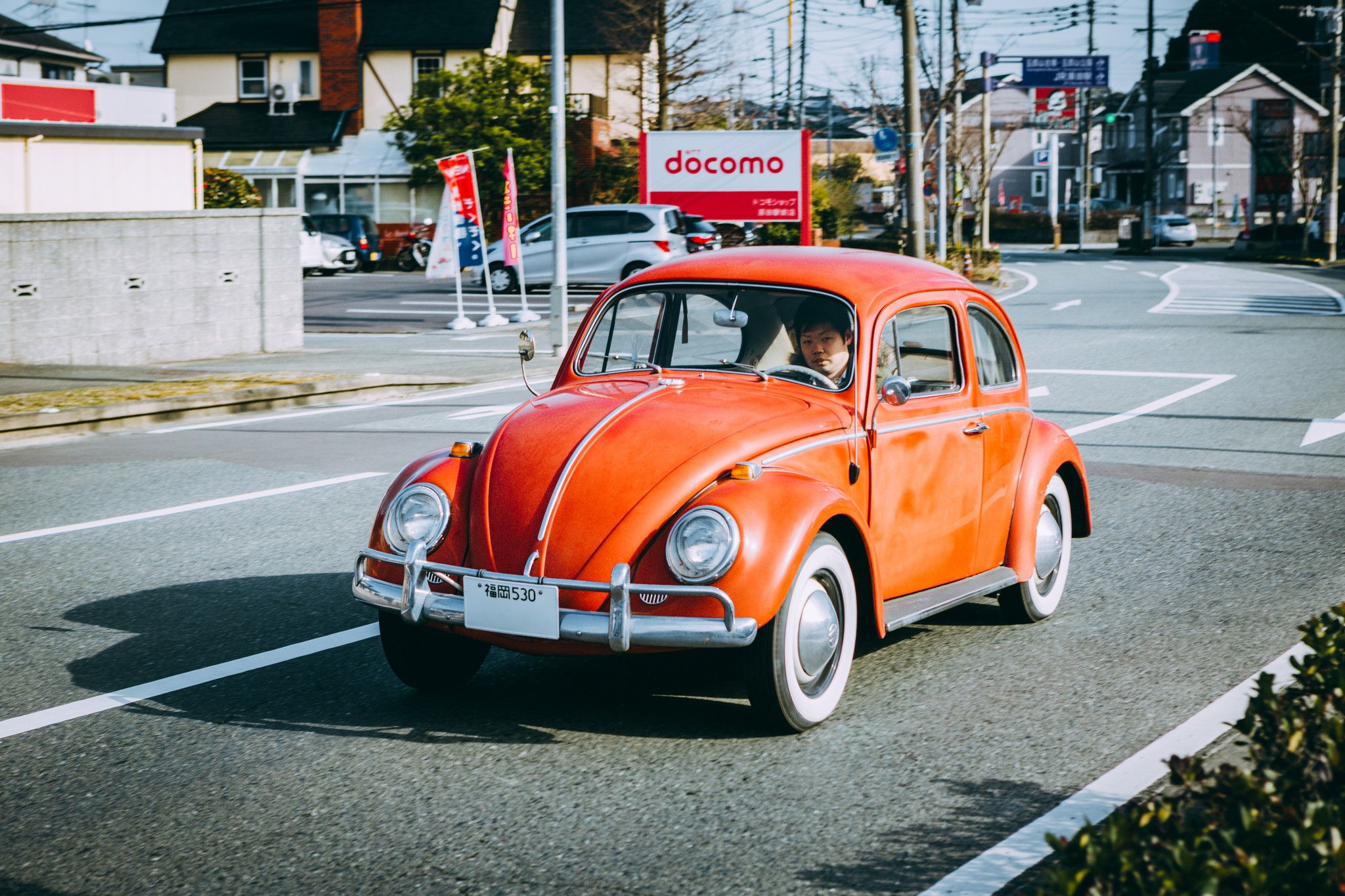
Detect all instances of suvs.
[305,210,383,272]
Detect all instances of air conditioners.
[269,81,301,102]
[85,71,130,85]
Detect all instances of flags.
[425,183,458,279]
[435,147,488,268]
[499,146,522,267]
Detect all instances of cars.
[1129,213,1198,249]
[350,240,1093,736]
[300,211,361,279]
[469,201,721,297]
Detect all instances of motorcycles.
[393,216,434,273]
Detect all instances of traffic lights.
[1105,112,1134,123]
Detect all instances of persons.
[1309,215,1323,255]
[768,298,856,392]
